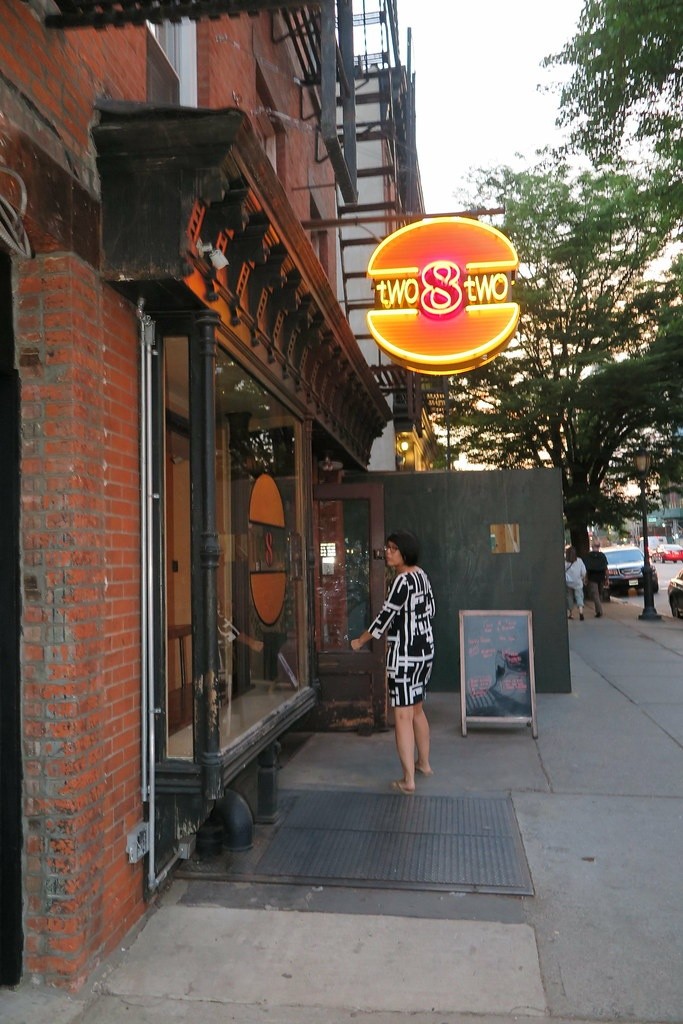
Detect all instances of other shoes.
[595,612,601,617]
[568,615,574,619]
[580,614,584,620]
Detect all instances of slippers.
[391,779,414,795]
[415,769,433,777]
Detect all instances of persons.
[564,545,586,620]
[584,541,609,618]
[351,529,436,795]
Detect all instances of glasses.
[383,545,399,554]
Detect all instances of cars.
[651,544,683,563]
[667,569,683,619]
[599,546,659,593]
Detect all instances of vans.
[639,537,668,557]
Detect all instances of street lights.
[631,444,664,619]
